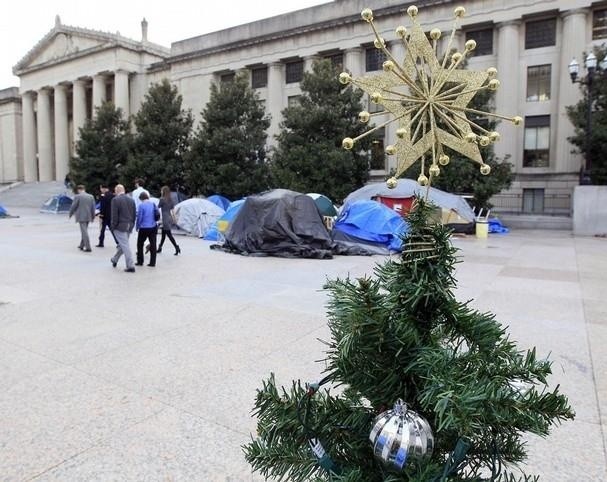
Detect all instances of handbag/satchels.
[154,203,160,221]
[170,209,177,224]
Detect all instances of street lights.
[569,51,607,185]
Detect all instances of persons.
[95,184,118,247]
[156,186,180,255]
[131,179,150,217]
[110,184,136,272]
[135,192,160,267]
[68,184,96,251]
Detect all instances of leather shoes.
[78,244,180,272]
